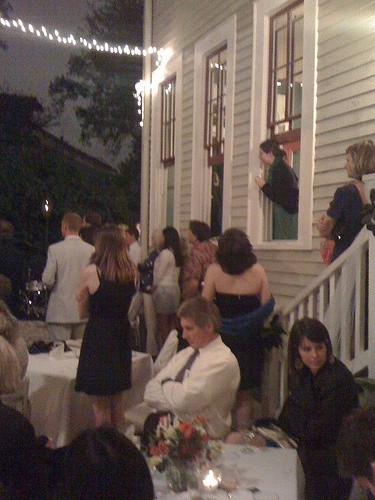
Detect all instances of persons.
[73,227,138,428]
[180,221,219,302]
[0,218,30,421]
[202,228,274,435]
[225,317,359,500]
[142,296,242,451]
[149,225,181,362]
[79,225,144,269]
[41,211,97,341]
[253,139,299,215]
[336,396,375,500]
[317,139,374,264]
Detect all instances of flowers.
[147,414,224,471]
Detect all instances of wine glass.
[219,462,241,500]
[238,414,255,454]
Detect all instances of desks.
[12,320,154,451]
[124,436,299,500]
